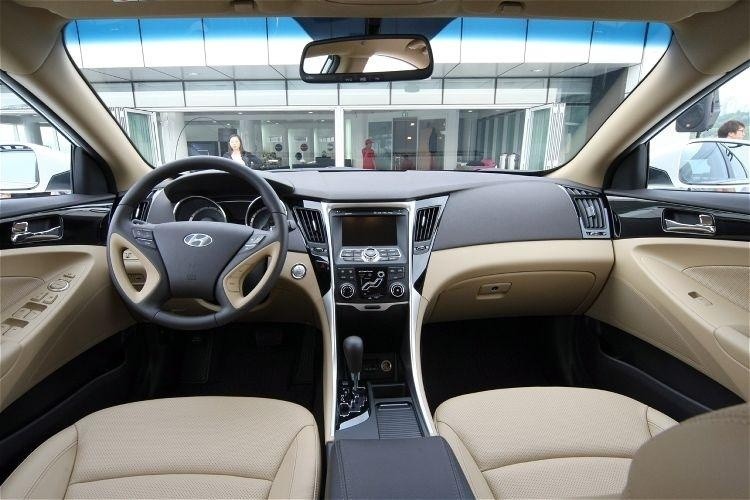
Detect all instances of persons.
[223,134,263,169]
[717,119,746,141]
[362,139,377,170]
[403,155,414,170]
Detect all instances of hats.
[365,140,374,145]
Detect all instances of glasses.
[737,129,744,134]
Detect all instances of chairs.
[434,386,750,500]
[0,397,322,500]
